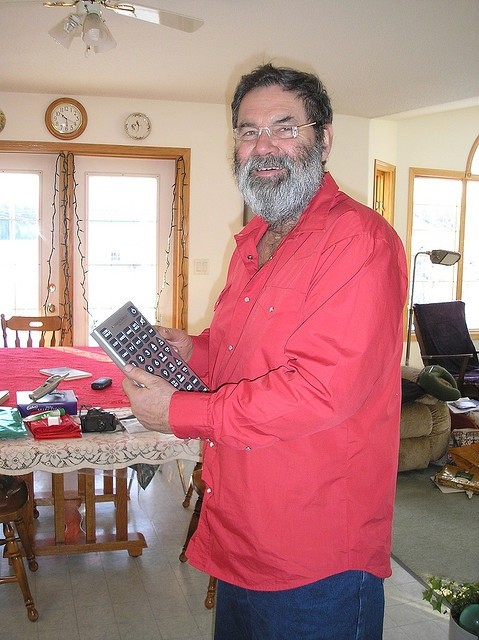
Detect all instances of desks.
[0,347,204,557]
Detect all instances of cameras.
[80,406,118,432]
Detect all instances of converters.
[48,415,60,427]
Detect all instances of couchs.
[398,366,452,473]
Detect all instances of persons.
[121,63,409,640]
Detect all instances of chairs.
[179,465,220,607]
[1,475,40,622]
[410,300,479,399]
[0,313,69,348]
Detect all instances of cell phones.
[26,373,69,402]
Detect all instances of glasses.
[232,121,316,141]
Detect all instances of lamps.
[44,1,206,34]
[405,249,461,366]
[42,10,116,55]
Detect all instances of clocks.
[44,98,87,139]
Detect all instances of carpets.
[390,472,479,608]
